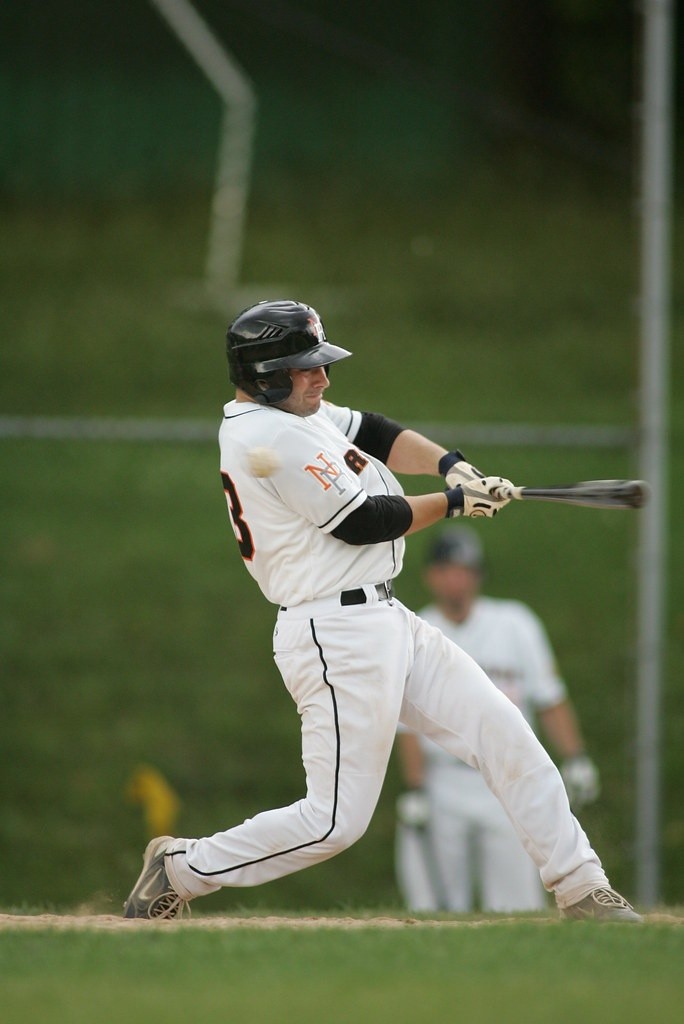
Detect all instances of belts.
[281,580,392,611]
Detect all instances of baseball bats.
[490,478,649,510]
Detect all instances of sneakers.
[559,887,642,923]
[123,836,191,919]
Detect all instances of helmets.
[226,299,353,404]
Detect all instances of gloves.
[560,755,600,805]
[395,789,429,827]
[439,449,514,519]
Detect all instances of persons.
[395,522,603,920]
[122,299,643,923]
